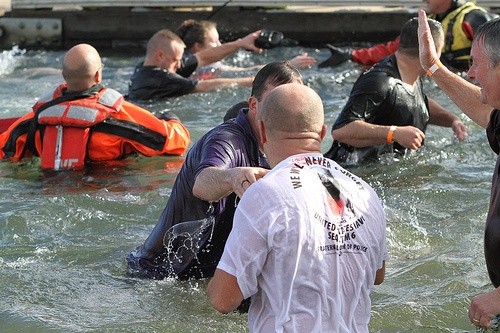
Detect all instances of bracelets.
[427,59,443,78]
[387,126,397,143]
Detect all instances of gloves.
[316,45,352,68]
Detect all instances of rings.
[241,180,250,188]
[473,318,479,323]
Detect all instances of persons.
[128,30,255,104]
[208,84,388,332]
[318,0,499,72]
[0,44,190,169]
[325,17,469,170]
[127,61,303,312]
[418,9,500,333]
[176,19,314,73]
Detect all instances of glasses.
[97,62,105,74]
